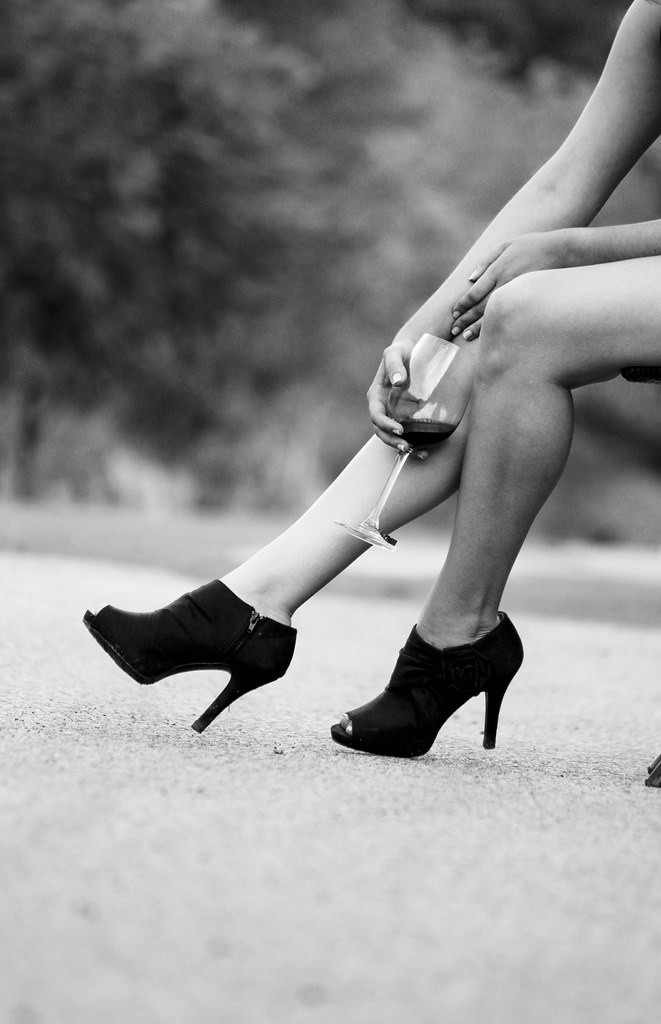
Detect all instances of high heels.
[331,611,524,759]
[84,579,298,734]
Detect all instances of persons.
[83,0,661,757]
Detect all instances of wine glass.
[333,331,476,553]
